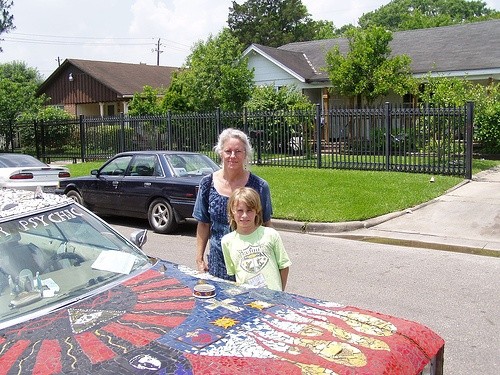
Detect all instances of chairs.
[136,163,151,174]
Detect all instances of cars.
[0,153,72,193]
[1,189,446,374]
[53,150,223,235]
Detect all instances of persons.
[192,128,273,282]
[221,187,292,292]
